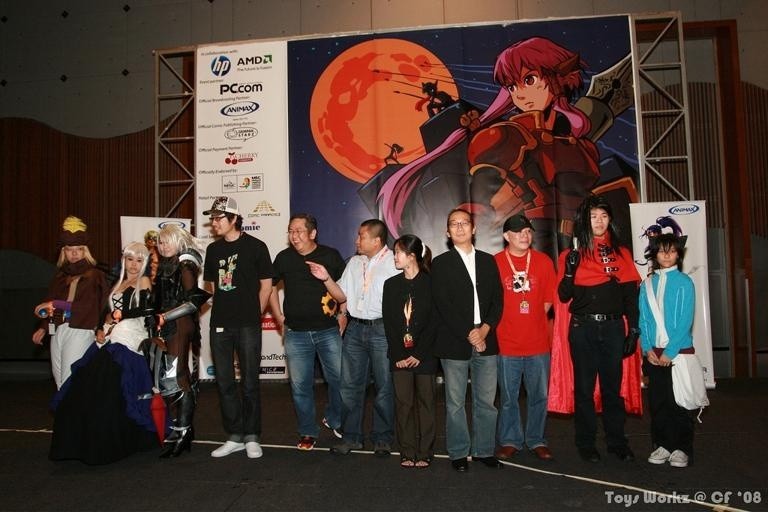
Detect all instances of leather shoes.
[496,446,519,458]
[374,439,392,456]
[532,447,553,461]
[471,455,503,469]
[452,458,468,472]
[331,432,364,454]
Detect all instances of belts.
[577,313,618,322]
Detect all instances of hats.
[202,197,240,216]
[503,215,536,233]
[58,217,87,247]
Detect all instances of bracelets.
[112,307,117,321]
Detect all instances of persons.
[270,214,347,452]
[430,209,506,473]
[382,234,443,468]
[379,37,602,238]
[31,215,110,393]
[50,242,153,464]
[492,214,557,462]
[199,197,273,460]
[304,219,405,459]
[151,224,212,459]
[639,235,696,468]
[548,196,642,466]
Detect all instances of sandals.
[400,457,415,469]
[415,457,431,470]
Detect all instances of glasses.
[209,216,227,222]
[449,221,470,228]
[286,231,307,235]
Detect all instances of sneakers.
[212,441,245,457]
[245,442,263,458]
[648,447,670,464]
[669,450,688,467]
[321,417,343,440]
[298,437,317,451]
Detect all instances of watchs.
[337,310,349,318]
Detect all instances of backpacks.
[646,271,711,414]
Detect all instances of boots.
[169,391,194,458]
[160,392,180,457]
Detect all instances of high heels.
[604,437,635,461]
[577,444,601,462]
